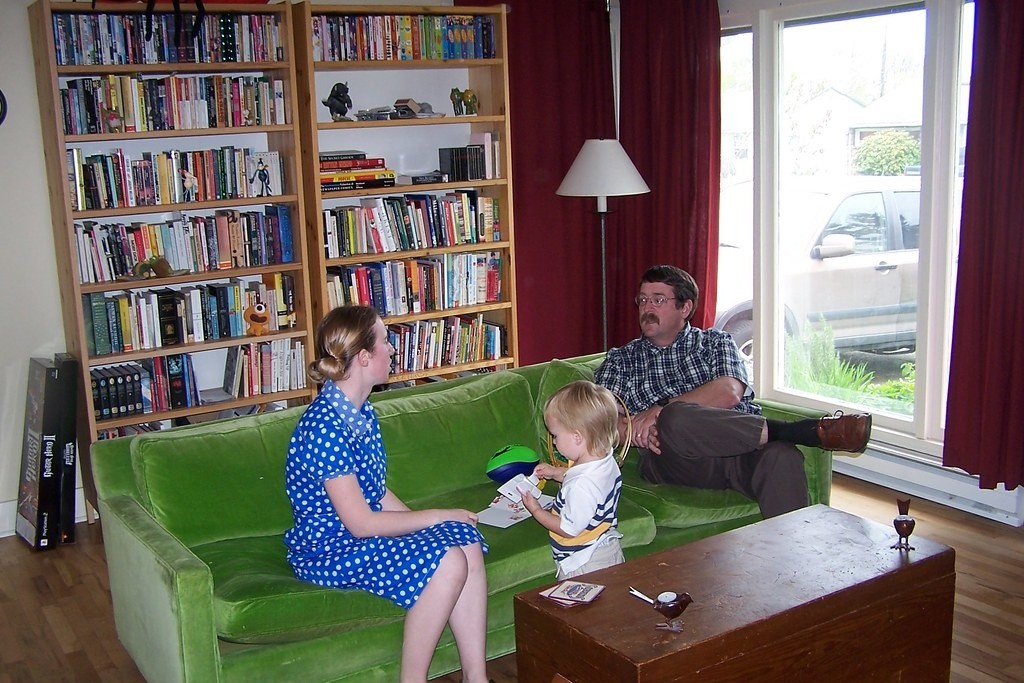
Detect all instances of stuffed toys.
[243,301,272,337]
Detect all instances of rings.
[636,433,641,438]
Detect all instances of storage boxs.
[15,357,65,553]
[54,352,81,546]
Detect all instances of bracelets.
[658,398,669,406]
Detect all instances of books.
[52,12,508,440]
[475,492,556,529]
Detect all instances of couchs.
[90,353,831,683]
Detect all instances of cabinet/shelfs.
[292,0,519,384]
[27,0,319,523]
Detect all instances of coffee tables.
[513,504,957,683]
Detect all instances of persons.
[592,264,872,520]
[520,379,625,581]
[283,304,495,683]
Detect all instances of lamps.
[555,138,652,352]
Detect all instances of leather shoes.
[816,409,872,451]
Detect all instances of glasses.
[634,293,676,308]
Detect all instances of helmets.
[485,444,541,484]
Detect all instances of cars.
[716,175,964,365]
